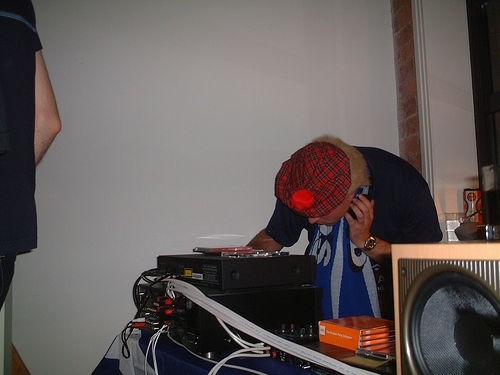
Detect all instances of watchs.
[360,234,377,252]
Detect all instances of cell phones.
[347,185,370,220]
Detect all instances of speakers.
[392,241,500,375]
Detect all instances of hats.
[274,143,351,218]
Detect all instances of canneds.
[463,188,484,225]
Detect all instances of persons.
[245,135,445,321]
[0,0,61,375]
